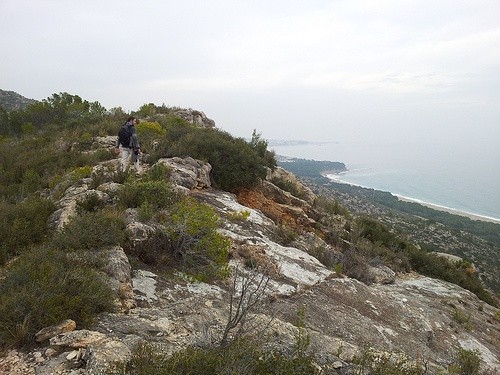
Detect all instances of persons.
[115,116,141,174]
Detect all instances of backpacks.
[118,124,134,147]
